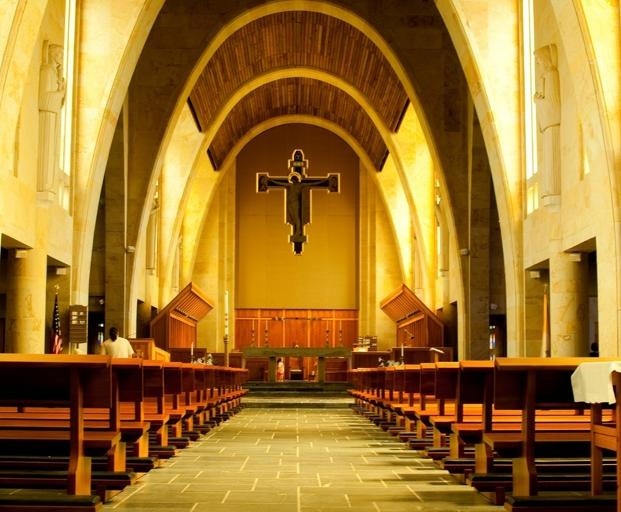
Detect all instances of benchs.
[0,350,250,511]
[347,354,621,511]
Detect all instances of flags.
[51,295,63,354]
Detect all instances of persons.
[527,45,563,196]
[37,43,65,192]
[377,355,385,367]
[277,358,284,380]
[268,175,327,236]
[100,327,135,358]
